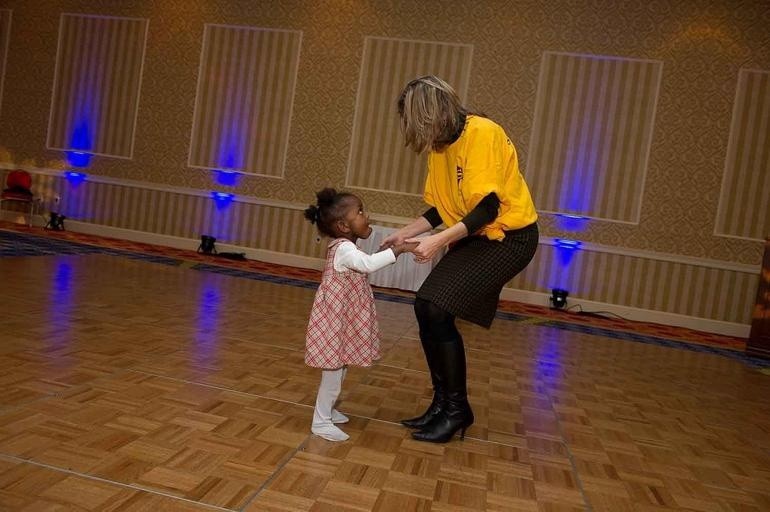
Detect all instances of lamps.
[45,210,66,230]
[197,234,218,256]
[550,287,569,312]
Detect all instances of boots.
[310,365,351,442]
[398,322,476,445]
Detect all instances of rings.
[418,258,422,262]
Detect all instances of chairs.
[0,169,38,228]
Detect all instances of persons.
[375,73,542,445]
[298,187,423,443]
